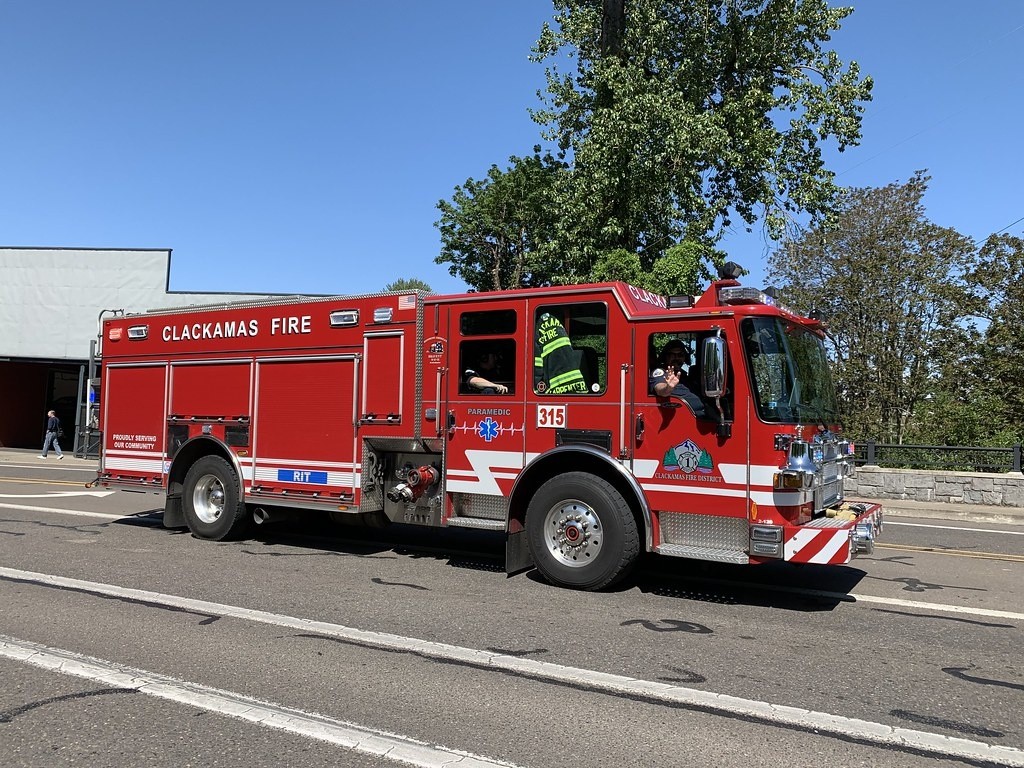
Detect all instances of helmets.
[479,341,502,356]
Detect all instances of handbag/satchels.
[57,417,64,438]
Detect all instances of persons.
[37,410,64,460]
[649,339,707,416]
[462,353,508,394]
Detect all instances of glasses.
[668,352,684,359]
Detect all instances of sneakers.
[57,454,64,460]
[37,455,47,460]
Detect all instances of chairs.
[572,347,600,394]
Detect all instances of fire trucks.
[73,280,884,591]
